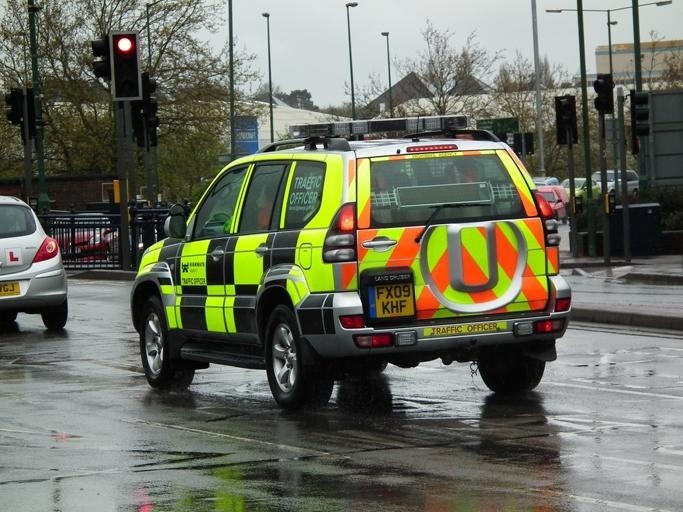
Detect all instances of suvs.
[131,129,573,405]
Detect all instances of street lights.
[260,9,274,144]
[546,1,673,198]
[345,0,360,118]
[381,31,393,116]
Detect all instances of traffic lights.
[90,30,144,105]
[5,86,23,127]
[133,72,161,148]
[591,70,614,114]
[628,88,650,155]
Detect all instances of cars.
[532,170,638,218]
[0,194,68,332]
[49,209,114,262]
